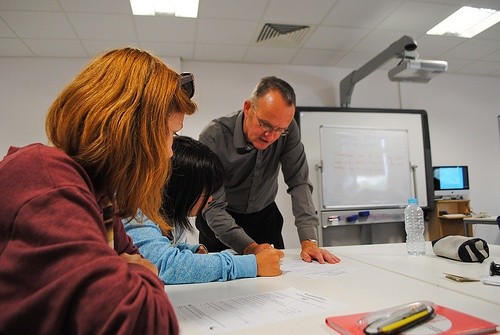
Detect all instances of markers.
[328,216,341,222]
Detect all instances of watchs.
[308,238,316,242]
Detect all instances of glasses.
[177,72,195,100]
[251,105,289,136]
[171,129,183,152]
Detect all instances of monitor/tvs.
[433,166,469,199]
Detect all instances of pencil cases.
[361,302,435,335]
[433,236,490,263]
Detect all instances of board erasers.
[346,214,358,222]
[358,211,370,217]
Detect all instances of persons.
[121,136,284,285]
[0,47,197,335]
[195,76,342,265]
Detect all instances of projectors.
[388,58,447,84]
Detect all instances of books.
[325,303,500,335]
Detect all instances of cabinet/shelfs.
[427,200,474,240]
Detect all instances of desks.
[463,216,500,238]
[162,242,500,335]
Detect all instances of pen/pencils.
[377,309,428,332]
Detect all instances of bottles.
[404,198,426,255]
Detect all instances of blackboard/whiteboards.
[319,124,415,208]
